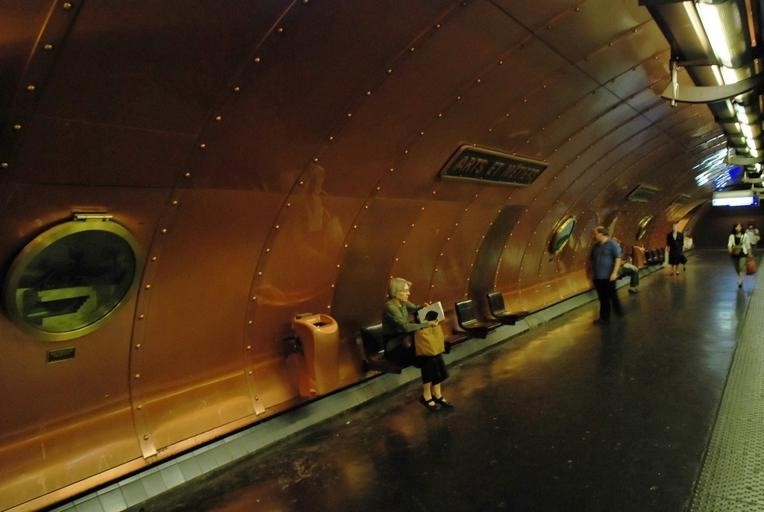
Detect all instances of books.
[417,301,445,324]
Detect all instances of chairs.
[644,247,665,265]
[361,322,420,373]
[437,292,528,354]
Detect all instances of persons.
[744,225,760,260]
[587,225,626,325]
[728,223,752,288]
[381,277,455,411]
[666,224,684,277]
[610,237,641,294]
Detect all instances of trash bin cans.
[633,245,647,269]
[292,312,340,399]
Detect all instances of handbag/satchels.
[415,325,445,357]
[732,246,742,254]
[745,257,755,273]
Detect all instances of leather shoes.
[419,395,440,410]
[592,317,610,325]
[432,395,452,407]
[671,271,679,275]
[629,287,640,294]
